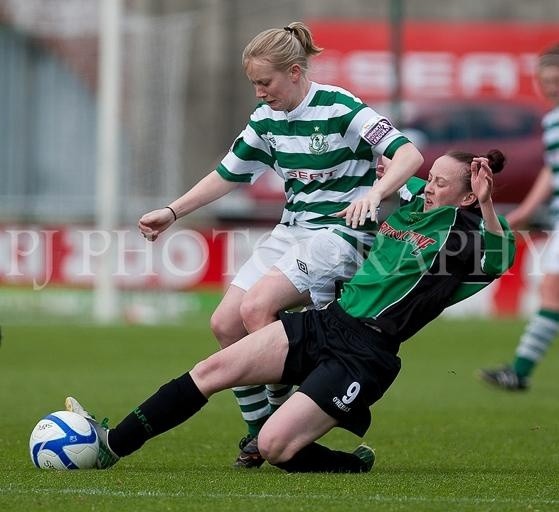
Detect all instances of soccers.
[29,410,100,470]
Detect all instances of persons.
[66,24,518,472]
[475,42,559,391]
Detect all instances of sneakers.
[235,435,266,469]
[476,368,531,393]
[65,396,119,471]
[353,441,377,474]
